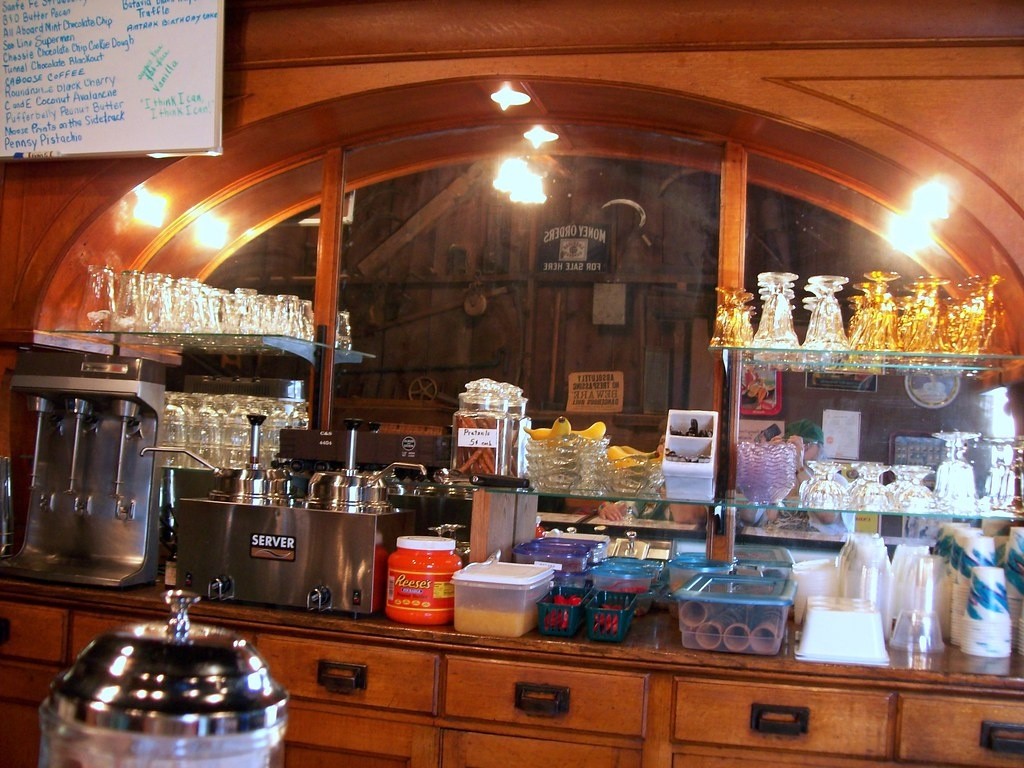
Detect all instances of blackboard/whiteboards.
[0,0,227,162]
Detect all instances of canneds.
[385,535,463,626]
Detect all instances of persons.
[770,418,854,534]
[598,421,710,524]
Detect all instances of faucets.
[359,463,428,513]
[139,447,234,501]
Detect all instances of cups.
[163,391,310,468]
[334,312,352,355]
[836,520,1024,657]
[792,559,836,624]
[78,263,315,354]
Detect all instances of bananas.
[608,443,663,467]
[522,416,606,440]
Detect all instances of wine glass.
[800,430,982,513]
[709,270,1012,373]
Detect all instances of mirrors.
[29,75,1023,452]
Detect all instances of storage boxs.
[449,535,798,656]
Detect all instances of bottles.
[385,536,463,625]
[502,382,531,477]
[448,378,506,486]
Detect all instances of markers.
[14,150,54,158]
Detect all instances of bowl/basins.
[735,441,797,506]
[512,537,607,588]
[668,546,798,655]
[522,434,665,495]
[589,555,665,615]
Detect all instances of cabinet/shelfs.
[0,582,1024,768]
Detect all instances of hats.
[782,418,824,445]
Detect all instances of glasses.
[804,441,818,452]
